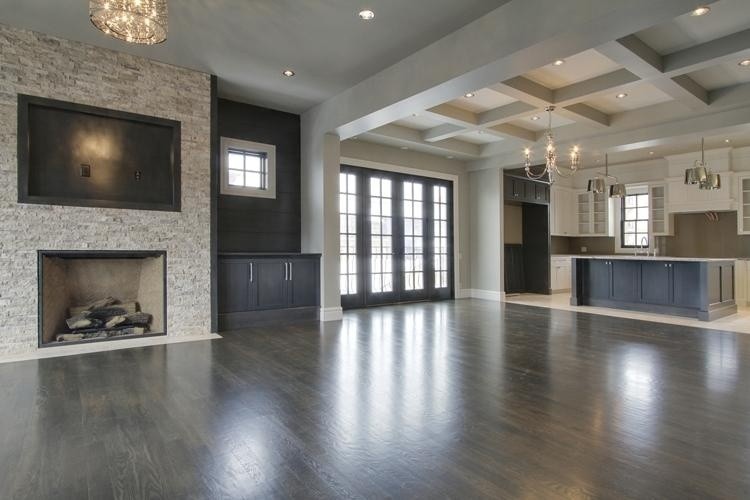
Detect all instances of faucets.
[641,237,647,251]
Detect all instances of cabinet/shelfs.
[253,258,320,312]
[551,256,571,293]
[575,193,613,237]
[582,259,638,311]
[736,176,750,236]
[639,261,737,322]
[649,184,675,237]
[219,258,253,314]
[665,171,738,214]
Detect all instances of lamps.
[523,106,582,183]
[89,1,169,47]
[586,153,626,199]
[684,136,721,190]
